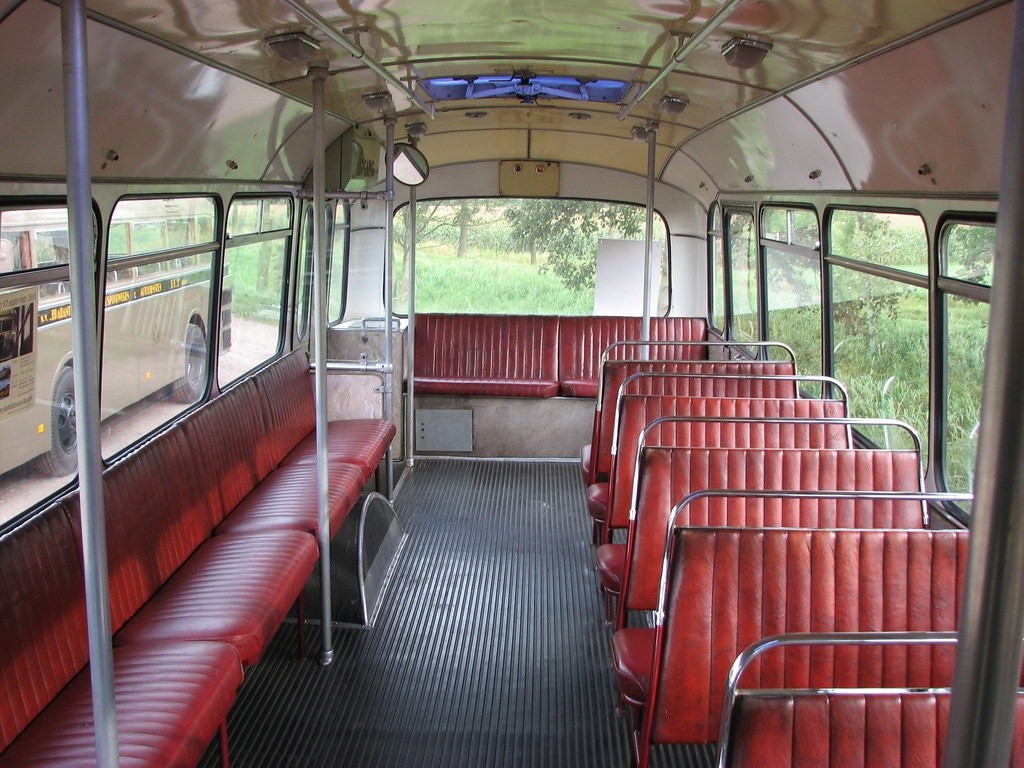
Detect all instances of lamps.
[362,92,394,115]
[721,38,770,69]
[631,128,649,142]
[658,97,689,114]
[266,33,319,64]
[405,120,427,138]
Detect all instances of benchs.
[0,312,1024,767]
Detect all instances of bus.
[0,205,234,483]
